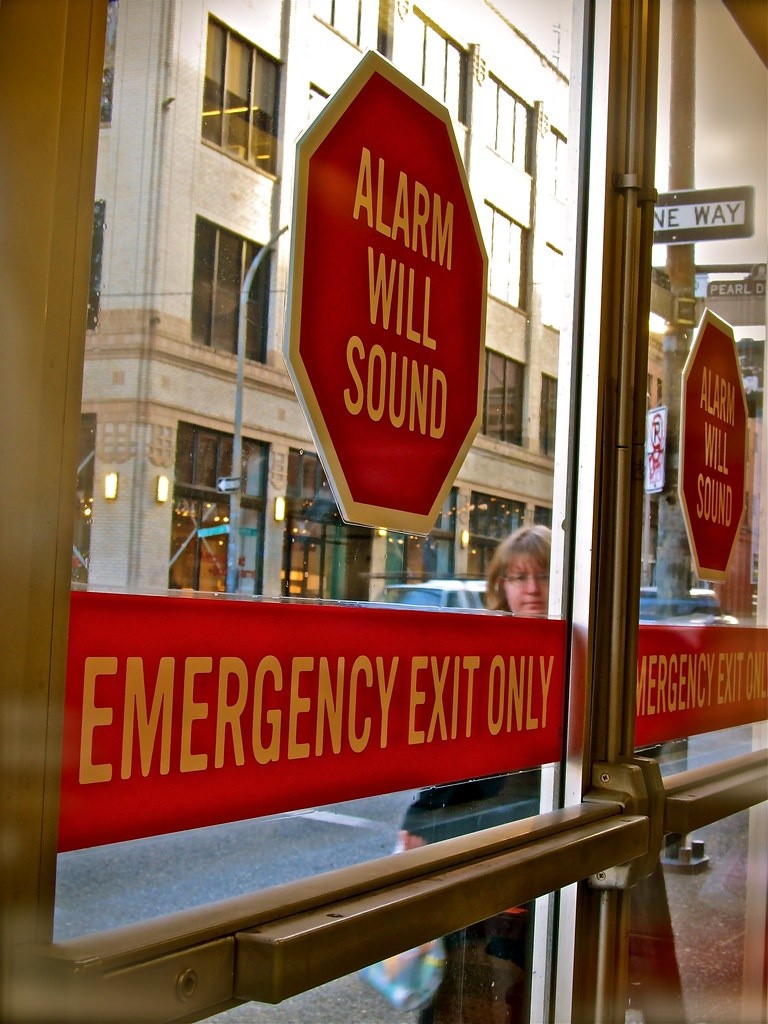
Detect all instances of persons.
[397,526,553,1024]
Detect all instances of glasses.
[500,571,549,589]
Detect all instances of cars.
[639,587,721,621]
[380,579,488,610]
